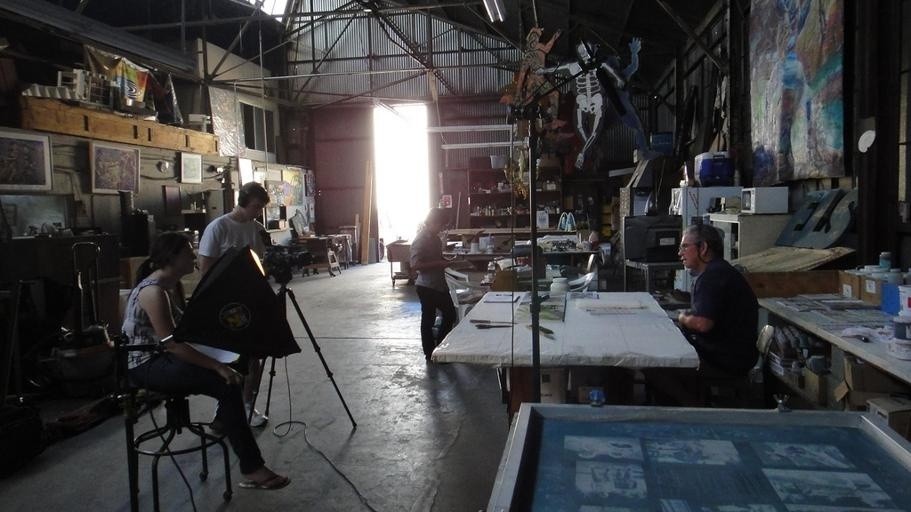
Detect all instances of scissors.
[476,324,513,329]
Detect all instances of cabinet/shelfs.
[0,232,126,367]
[466,154,566,230]
[701,208,795,298]
[618,184,656,234]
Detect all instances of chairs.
[110,329,235,511]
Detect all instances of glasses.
[679,243,699,252]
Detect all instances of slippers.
[238,472,294,490]
[209,419,229,435]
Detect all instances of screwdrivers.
[470,320,519,324]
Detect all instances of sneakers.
[424,355,451,366]
[242,403,269,426]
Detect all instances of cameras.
[261,244,295,284]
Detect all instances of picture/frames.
[180,149,203,184]
[0,128,54,196]
[87,139,140,196]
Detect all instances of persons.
[120,231,290,490]
[409,209,458,366]
[199,181,274,435]
[642,223,760,407]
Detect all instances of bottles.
[550,278,571,292]
[567,216,574,231]
[541,179,557,191]
[684,161,695,186]
[545,200,561,214]
[472,202,496,217]
[561,214,567,230]
[887,269,903,286]
[586,230,600,249]
[880,251,891,271]
[487,262,495,275]
[903,268,911,285]
[546,264,552,280]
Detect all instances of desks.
[384,240,535,289]
[443,226,577,242]
[620,258,688,296]
[428,291,703,430]
[332,233,353,270]
[486,400,910,511]
[295,234,342,277]
[755,291,909,444]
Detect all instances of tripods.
[247,289,356,440]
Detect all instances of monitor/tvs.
[622,213,683,264]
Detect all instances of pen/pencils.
[857,336,869,343]
[495,294,513,296]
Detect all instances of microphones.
[576,40,627,117]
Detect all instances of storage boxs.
[693,149,736,187]
[772,333,909,438]
[833,263,908,316]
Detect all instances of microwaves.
[742,188,790,215]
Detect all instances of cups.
[471,242,479,254]
[486,238,495,252]
[489,154,507,169]
